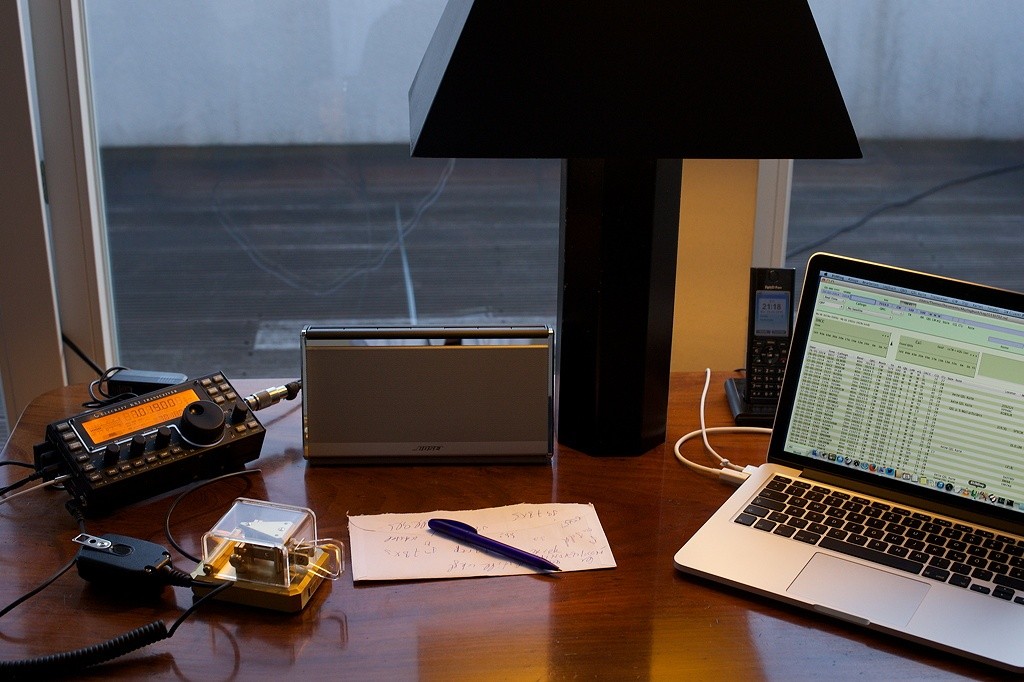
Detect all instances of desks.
[0,371,1009,681]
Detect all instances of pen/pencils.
[428,518,563,572]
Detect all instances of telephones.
[725,266,798,425]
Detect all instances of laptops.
[673,251,1024,675]
